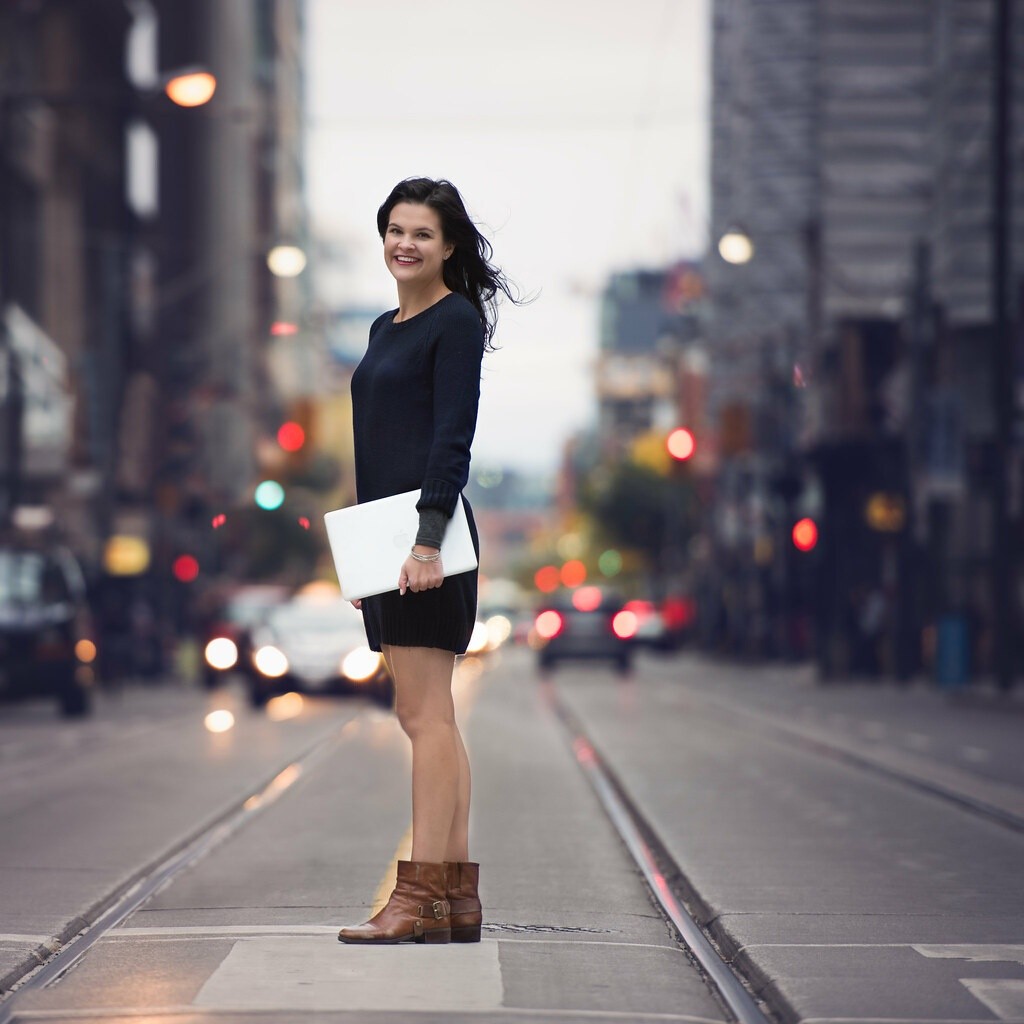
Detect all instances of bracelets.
[410,545,441,563]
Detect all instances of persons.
[338,178,501,943]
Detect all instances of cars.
[463,579,684,673]
[253,593,394,712]
[0,532,100,720]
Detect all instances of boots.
[336,858,484,945]
